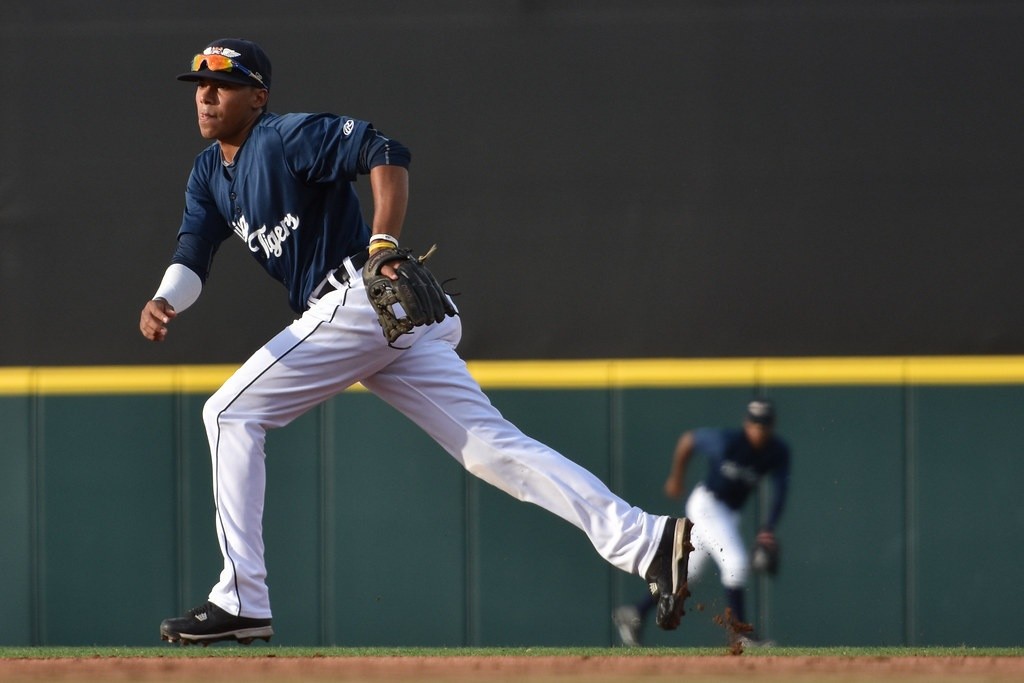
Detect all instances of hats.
[747,399,775,424]
[176,38,272,90]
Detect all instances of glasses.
[191,53,267,88]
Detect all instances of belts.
[306,251,370,310]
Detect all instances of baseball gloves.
[361,246,458,345]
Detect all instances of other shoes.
[735,629,773,649]
[612,605,641,647]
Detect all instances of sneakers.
[157,601,274,645]
[646,517,691,629]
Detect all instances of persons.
[137,36,697,648]
[611,399,795,656]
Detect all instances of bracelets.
[368,234,400,253]
